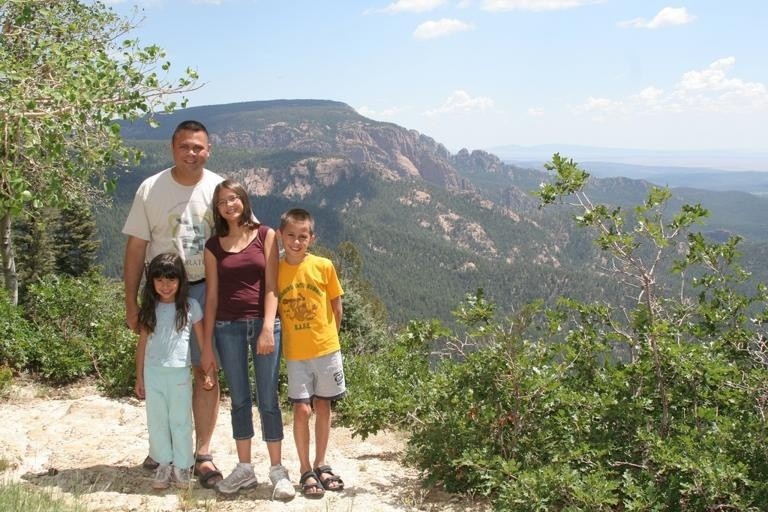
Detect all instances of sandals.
[144,456,172,468]
[191,452,223,489]
[300,470,325,498]
[313,462,344,490]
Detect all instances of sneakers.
[152,464,173,489]
[215,463,258,497]
[268,465,296,502]
[171,466,192,489]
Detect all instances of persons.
[122,122,260,488]
[199,179,295,499]
[135,253,215,490]
[276,208,345,495]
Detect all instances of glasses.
[215,194,241,209]
